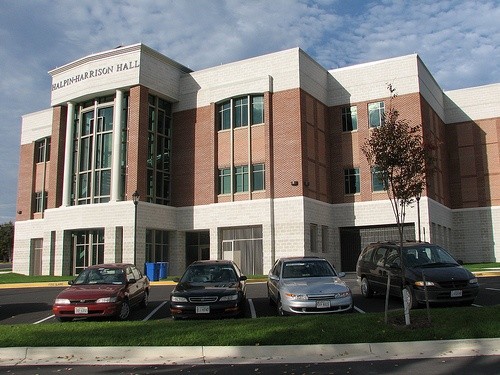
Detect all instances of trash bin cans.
[157,261,169,280]
[145,262,160,282]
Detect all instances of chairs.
[420,252,431,262]
[406,254,416,264]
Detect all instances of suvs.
[355,239,480,311]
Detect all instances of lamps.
[18,211,22,214]
[304,181,309,186]
[291,181,298,187]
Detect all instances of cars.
[169,259,248,321]
[52,263,150,323]
[267,257,353,316]
